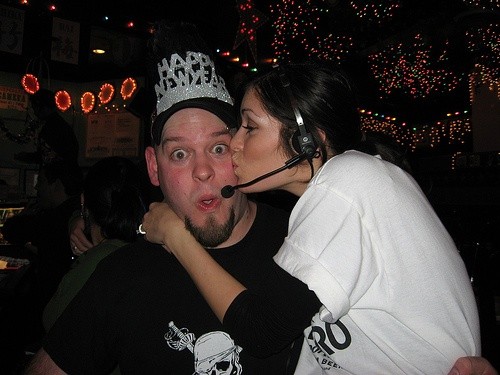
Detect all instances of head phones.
[274,65,321,169]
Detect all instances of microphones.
[221,166,288,199]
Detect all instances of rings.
[73,246,78,250]
[136,224,146,234]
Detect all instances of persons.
[28,90,80,194]
[66,64,481,375]
[3,167,89,268]
[22,73,497,375]
